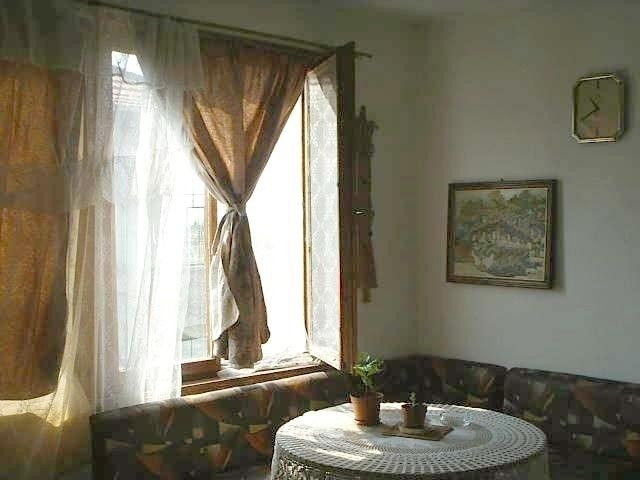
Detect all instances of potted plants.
[401,392,427,430]
[349,351,382,425]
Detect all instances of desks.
[271,403,548,480]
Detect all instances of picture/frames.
[445,179,556,288]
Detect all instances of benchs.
[90,356,640,480]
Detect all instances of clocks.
[570,72,625,143]
